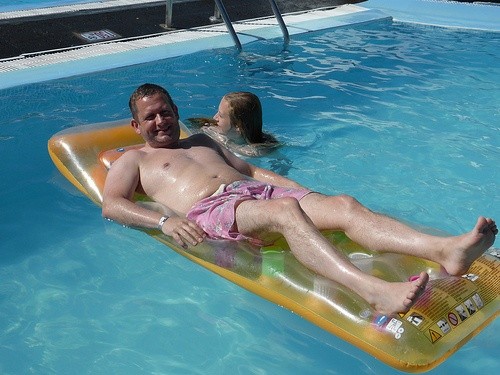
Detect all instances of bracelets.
[158,216,168,231]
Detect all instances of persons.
[184,92,296,176]
[101,83,499,315]
